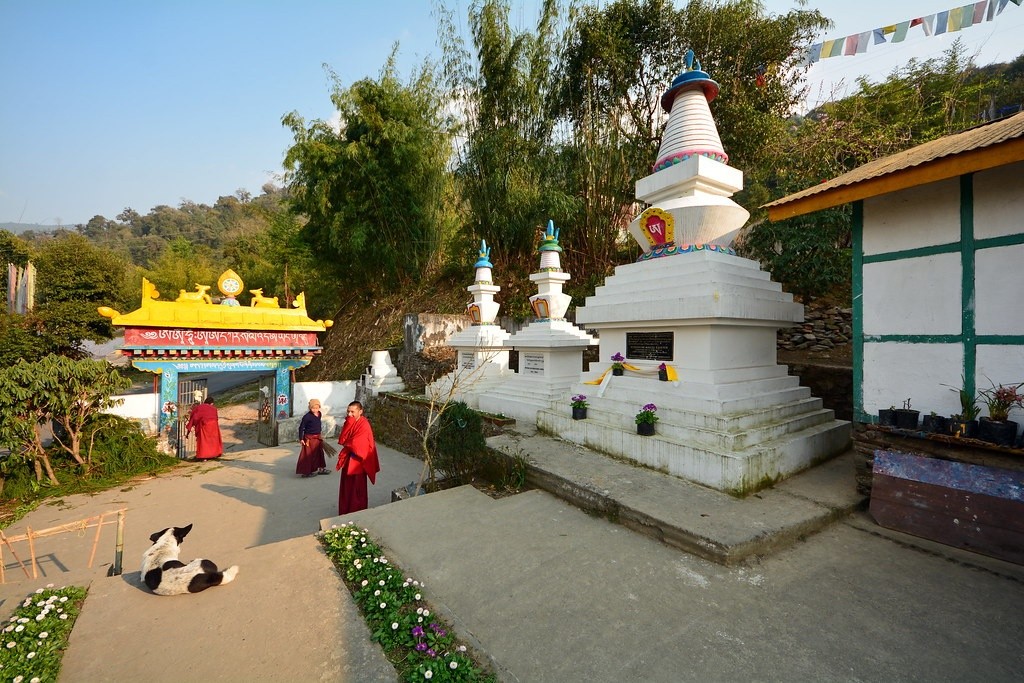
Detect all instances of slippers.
[318,469,331,475]
[302,473,317,477]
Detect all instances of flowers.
[976,374,1024,419]
[658,362,667,371]
[569,395,590,408]
[634,403,659,425]
[610,351,624,370]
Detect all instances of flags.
[795,0,1024,66]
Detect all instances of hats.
[309,399,321,411]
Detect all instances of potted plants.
[878,406,896,426]
[938,374,993,437]
[923,412,944,433]
[897,398,920,429]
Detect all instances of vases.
[572,407,586,420]
[979,417,1019,446]
[659,371,668,381]
[614,368,623,376]
[636,422,656,436]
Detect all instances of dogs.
[140,523,239,596]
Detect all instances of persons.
[296,399,331,478]
[185,398,222,461]
[336,401,380,515]
